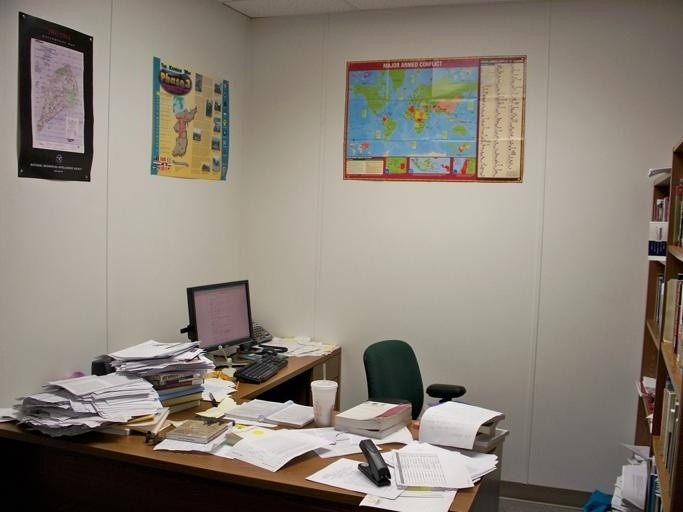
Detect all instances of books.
[607,164,683,511]
[2,336,506,512]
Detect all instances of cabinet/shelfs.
[627,141,682,510]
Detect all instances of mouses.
[262,348,277,356]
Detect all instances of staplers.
[358,440,392,486]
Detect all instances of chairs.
[361,339,466,421]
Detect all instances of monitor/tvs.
[187,280,253,370]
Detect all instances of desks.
[0,339,501,510]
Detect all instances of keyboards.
[233,355,287,384]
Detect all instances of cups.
[310,379,338,428]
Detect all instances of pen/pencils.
[209,393,218,407]
